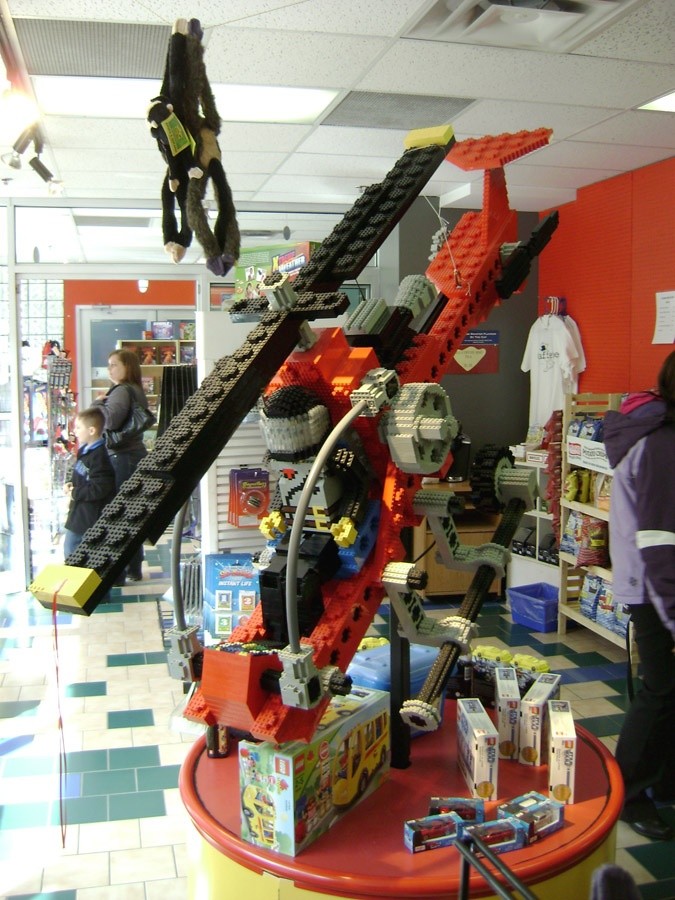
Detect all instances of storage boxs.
[238,683,391,858]
[457,699,498,801]
[142,331,153,340]
[500,791,547,820]
[506,582,571,633]
[345,641,447,735]
[519,673,561,766]
[180,346,194,364]
[139,346,157,365]
[428,797,485,826]
[545,699,577,805]
[513,800,565,846]
[495,668,522,760]
[180,322,195,340]
[158,346,176,365]
[146,321,174,340]
[403,811,465,855]
[526,450,550,463]
[141,376,155,394]
[462,817,524,859]
[235,240,322,302]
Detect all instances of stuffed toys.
[146,17,239,277]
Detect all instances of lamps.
[0,124,35,169]
[138,279,148,293]
[29,156,67,198]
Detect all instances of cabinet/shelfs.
[505,460,582,607]
[413,481,502,602]
[557,391,627,650]
[114,339,197,425]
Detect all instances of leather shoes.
[629,806,672,840]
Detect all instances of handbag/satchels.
[102,383,157,448]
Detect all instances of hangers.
[546,296,558,317]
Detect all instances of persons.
[64,408,115,604]
[604,352,675,840]
[89,349,148,580]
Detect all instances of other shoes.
[126,572,143,582]
[113,578,126,585]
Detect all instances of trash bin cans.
[508,582,558,633]
